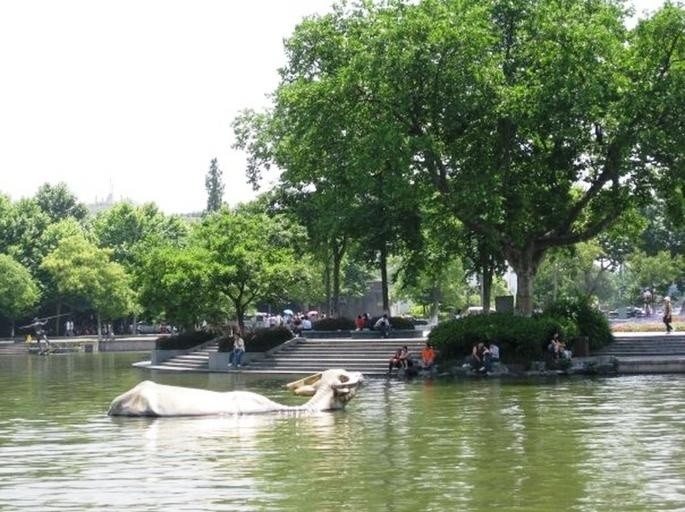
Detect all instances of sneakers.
[462,363,471,368]
[666,328,674,334]
[480,367,485,372]
[228,364,241,368]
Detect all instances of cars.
[610,306,643,317]
[128,320,172,334]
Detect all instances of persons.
[19,315,50,352]
[225,332,246,368]
[455,308,462,320]
[641,290,653,315]
[551,331,567,360]
[417,342,436,370]
[478,340,500,374]
[662,296,675,335]
[646,288,656,314]
[471,339,489,373]
[399,344,413,370]
[385,348,402,376]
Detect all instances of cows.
[106,368,366,417]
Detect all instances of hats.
[664,296,670,301]
